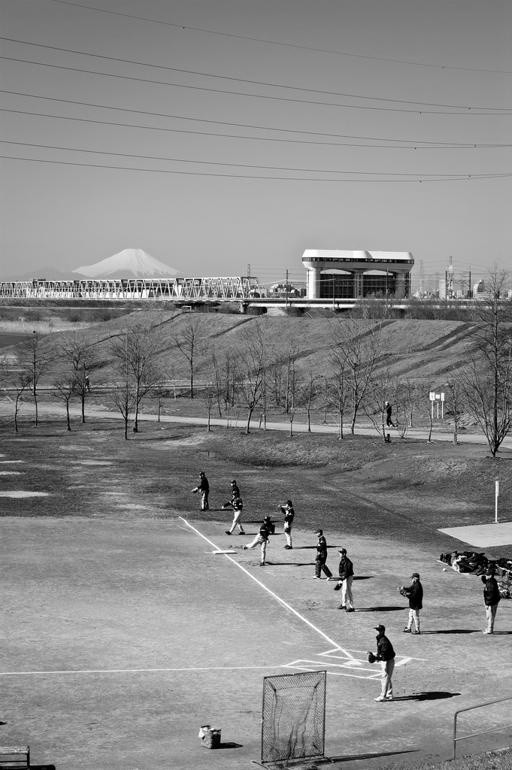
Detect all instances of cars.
[268,283,300,298]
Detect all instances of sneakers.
[243,544,333,582]
[336,604,357,613]
[373,694,394,702]
[402,626,422,636]
[224,530,245,536]
[483,628,493,634]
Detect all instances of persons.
[221,491,245,536]
[312,528,333,581]
[229,480,241,521]
[333,548,356,612]
[397,573,424,635]
[480,567,502,635]
[366,624,395,702]
[277,499,294,550]
[384,400,396,427]
[191,470,210,512]
[242,515,276,566]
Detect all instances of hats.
[284,500,292,505]
[338,548,347,553]
[229,479,237,495]
[263,516,270,522]
[197,471,204,476]
[408,571,420,579]
[373,623,386,631]
[485,569,495,575]
[314,529,323,534]
